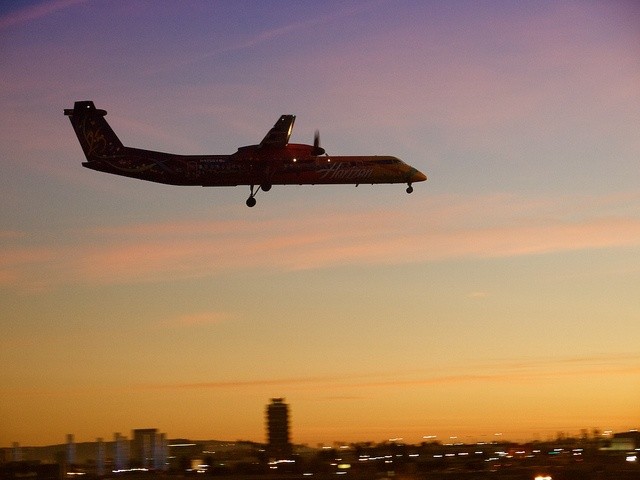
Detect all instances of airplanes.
[60,99,428,209]
